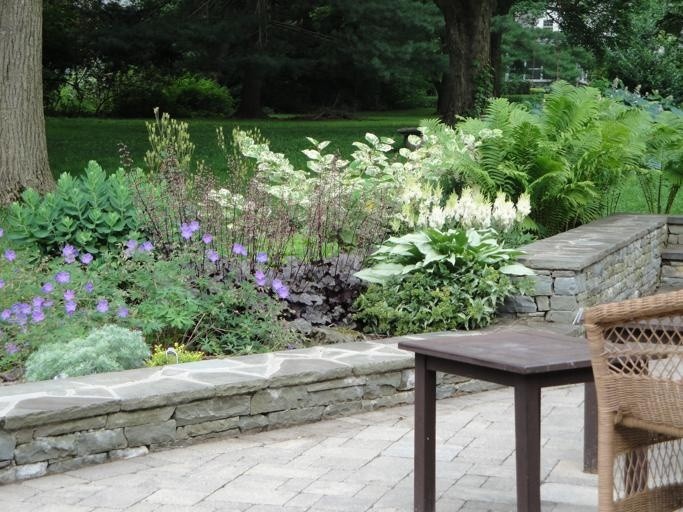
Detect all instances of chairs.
[583,289,683,512]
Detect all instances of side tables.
[398,328,668,511]
